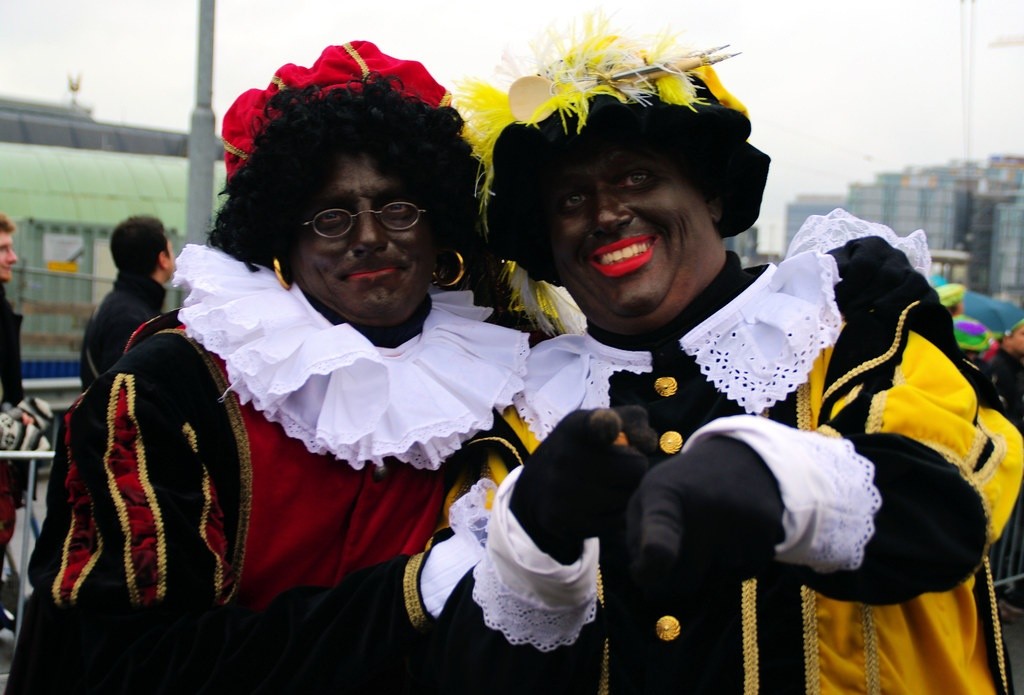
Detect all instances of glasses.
[302,202,426,238]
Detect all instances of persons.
[1,39,565,695]
[416,14,1024,695]
[928,273,1024,616]
[77,212,175,393]
[0,212,33,645]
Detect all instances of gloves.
[508,405,658,566]
[0,397,54,451]
[625,437,785,578]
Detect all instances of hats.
[221,40,449,185]
[454,37,771,338]
